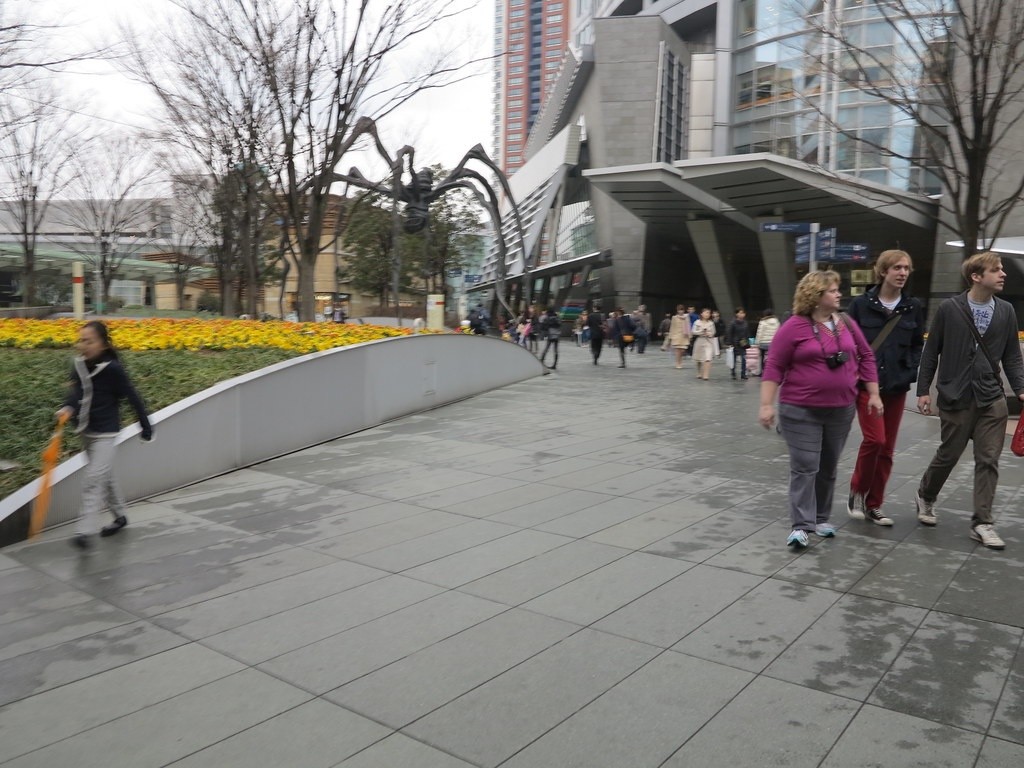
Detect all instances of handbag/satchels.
[726,347,734,369]
[1011,409,1024,456]
[623,335,633,342]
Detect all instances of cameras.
[825,351,849,370]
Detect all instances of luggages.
[746,348,761,376]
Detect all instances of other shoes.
[697,375,702,378]
[660,347,666,351]
[703,374,708,379]
[68,535,94,550]
[617,365,625,368]
[742,373,748,379]
[732,372,736,379]
[101,515,127,537]
[638,351,644,353]
[675,366,683,368]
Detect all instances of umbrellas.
[29,412,71,540]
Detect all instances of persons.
[333,303,344,323]
[754,309,781,377]
[514,303,562,370]
[724,307,751,380]
[759,270,884,547]
[657,302,724,380]
[846,250,925,526]
[470,303,490,334]
[53,320,154,552]
[916,252,1024,549]
[575,304,651,369]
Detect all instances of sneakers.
[817,522,837,538]
[847,493,866,519]
[866,508,894,525]
[786,529,810,547]
[915,492,937,524]
[970,523,1006,549]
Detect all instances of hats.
[666,314,670,316]
